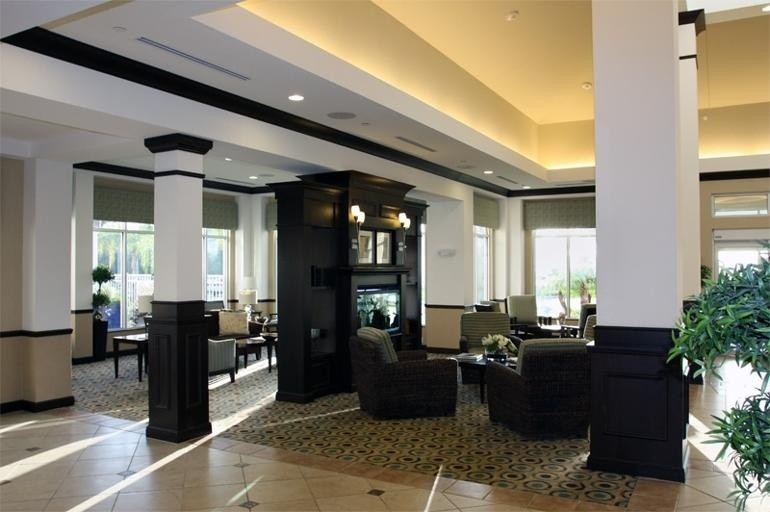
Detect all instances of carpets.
[71,350,638,507]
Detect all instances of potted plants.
[90,265,115,361]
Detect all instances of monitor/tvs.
[355,285,400,336]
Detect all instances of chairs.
[348,294,596,438]
[205,300,278,383]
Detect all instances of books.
[456,352,483,360]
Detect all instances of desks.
[112,333,149,382]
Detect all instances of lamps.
[238,290,256,321]
[351,204,365,264]
[398,212,411,265]
